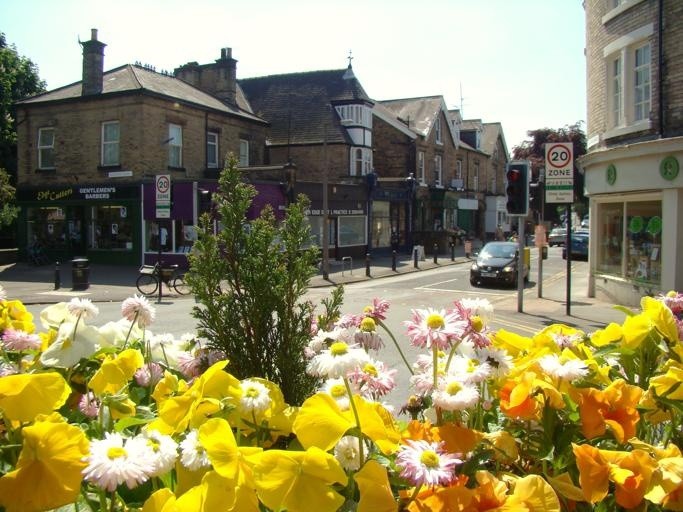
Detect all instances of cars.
[562,230,589,262]
[469,240,531,290]
[548,228,574,247]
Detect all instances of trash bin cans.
[70,258,89,290]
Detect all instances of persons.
[508,231,519,243]
[30,241,52,265]
[494,224,505,242]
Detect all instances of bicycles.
[136,260,194,296]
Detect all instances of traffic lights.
[504,160,529,218]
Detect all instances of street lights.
[192,181,210,294]
[365,172,378,277]
[283,156,297,215]
[321,118,354,280]
[404,172,419,268]
